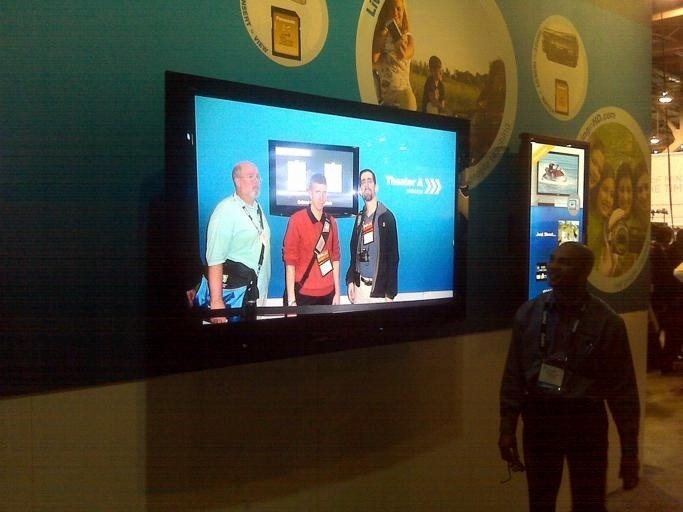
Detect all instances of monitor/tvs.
[267,139,360,220]
[512,131,591,313]
[160,68,470,378]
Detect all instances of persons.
[371,0,418,112]
[344,168,399,304]
[495,240,642,511]
[588,140,650,277]
[280,172,340,318]
[647,223,683,373]
[421,55,446,116]
[205,160,274,324]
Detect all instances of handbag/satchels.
[194,258,259,323]
[283,283,301,317]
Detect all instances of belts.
[361,277,372,286]
